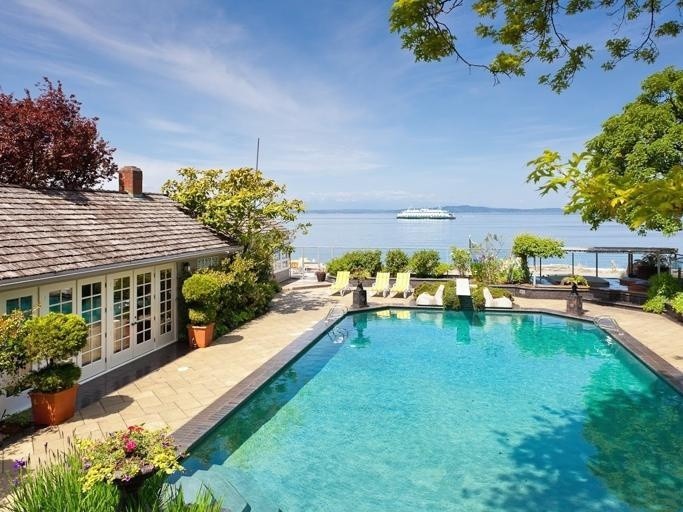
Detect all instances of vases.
[115,467,158,494]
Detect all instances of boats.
[395,205,456,219]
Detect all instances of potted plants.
[635,254,670,275]
[559,275,589,293]
[181,275,218,347]
[23,311,89,427]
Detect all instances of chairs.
[417,285,444,306]
[329,271,413,299]
[484,287,513,308]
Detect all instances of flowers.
[81,423,183,489]
